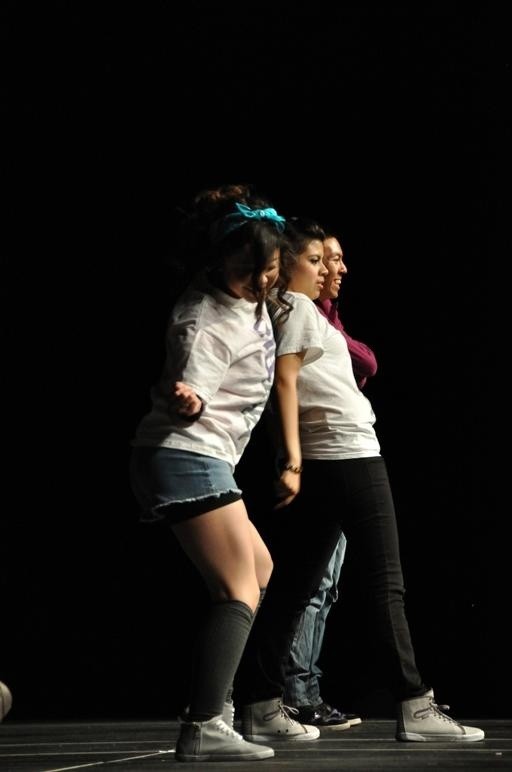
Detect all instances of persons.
[240,214,488,744]
[124,184,298,761]
[284,233,379,731]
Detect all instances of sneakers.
[318,698,364,727]
[393,686,486,742]
[284,702,350,732]
[238,693,321,744]
[174,695,238,732]
[173,711,276,762]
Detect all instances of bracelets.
[180,394,203,422]
[283,461,303,474]
